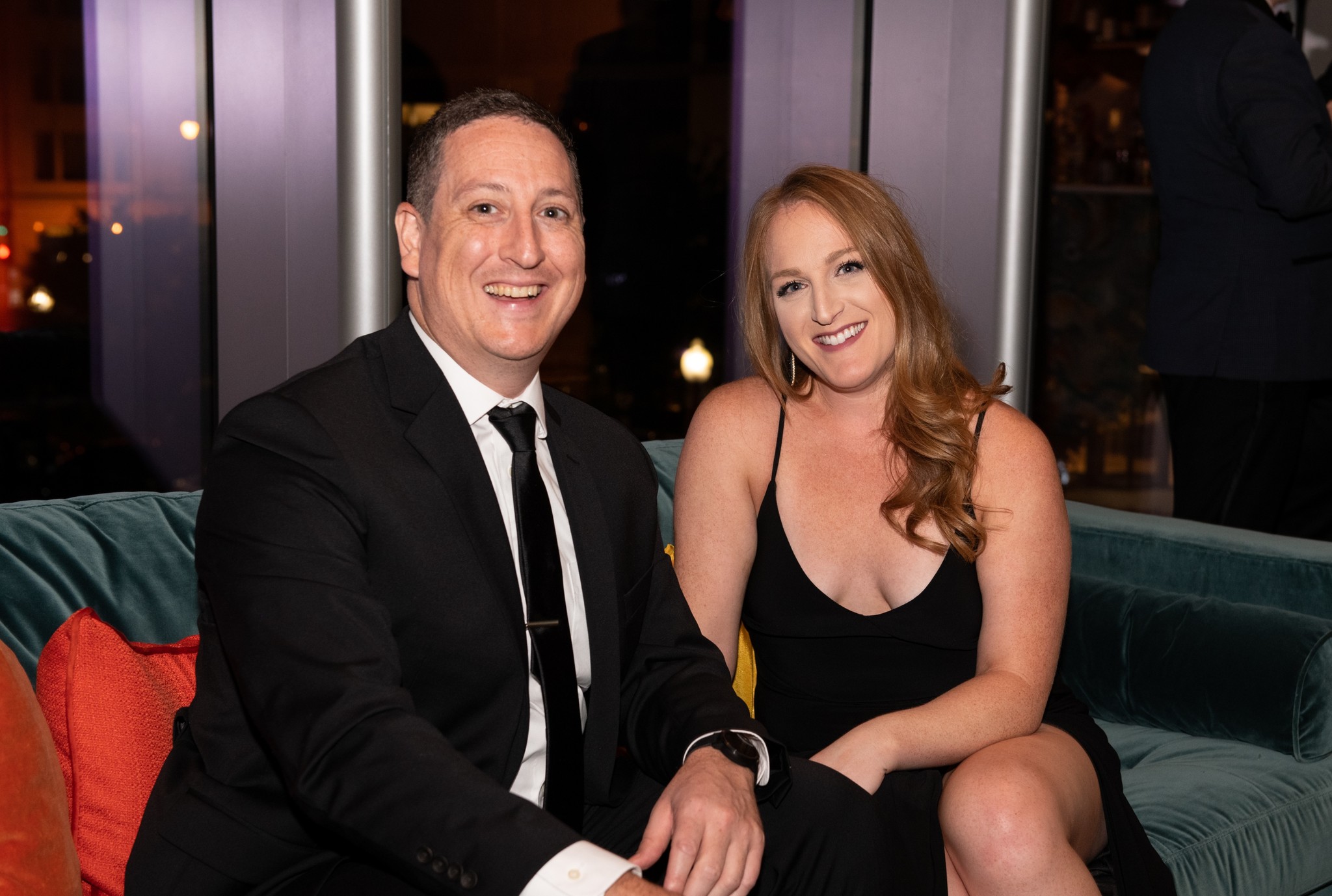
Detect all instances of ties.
[487,401,584,819]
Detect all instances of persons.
[661,161,1182,896]
[120,87,904,896]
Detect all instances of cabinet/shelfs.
[1035,0,1171,488]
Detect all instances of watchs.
[685,729,761,774]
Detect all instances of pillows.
[37,607,202,896]
[0,641,85,896]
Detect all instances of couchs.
[0,439,1331,896]
